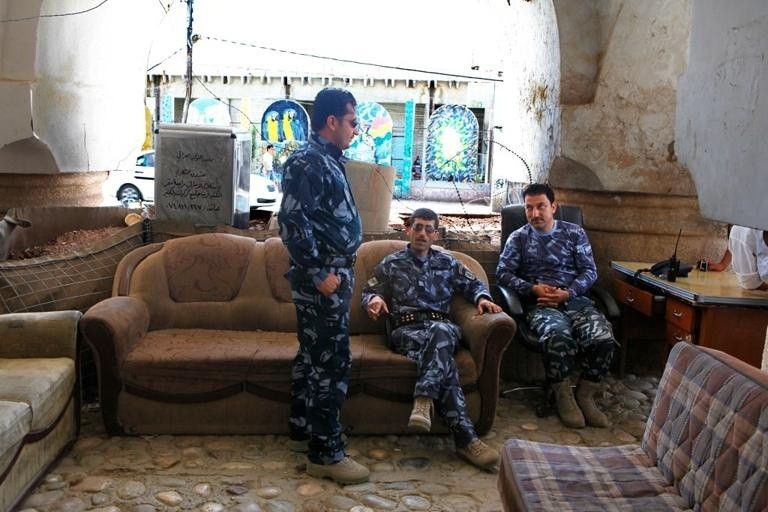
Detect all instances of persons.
[263,145,275,181]
[360,208,502,470]
[279,86,370,485]
[700,226,733,272]
[727,225,768,373]
[496,184,615,429]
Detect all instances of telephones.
[650,259,693,277]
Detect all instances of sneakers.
[405,394,435,436]
[304,451,371,486]
[289,433,350,455]
[458,436,503,468]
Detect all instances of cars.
[106,150,280,211]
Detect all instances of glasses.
[411,223,438,234]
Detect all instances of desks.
[609,259,767,379]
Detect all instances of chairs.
[501,205,623,419]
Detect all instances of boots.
[551,375,608,428]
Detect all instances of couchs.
[73,232,517,439]
[1,311,85,512]
[497,341,766,512]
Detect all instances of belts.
[396,309,450,324]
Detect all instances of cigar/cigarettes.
[369,308,379,317]
